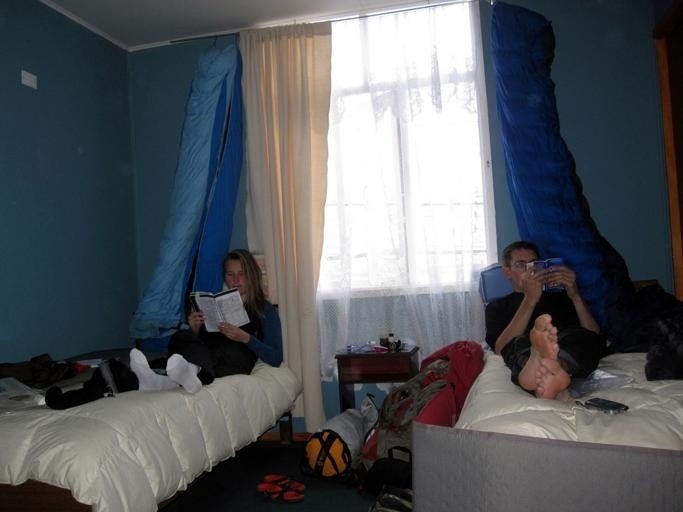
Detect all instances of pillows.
[219,248,269,302]
[478,257,564,353]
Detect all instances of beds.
[410,279,682,512]
[0,358,302,511]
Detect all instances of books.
[528,260,571,293]
[188,286,252,334]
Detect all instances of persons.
[483,241,607,401]
[129,246,284,393]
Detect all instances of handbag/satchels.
[363,446,412,494]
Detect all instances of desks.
[335,345,419,415]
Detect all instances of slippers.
[255,482,304,504]
[264,474,305,492]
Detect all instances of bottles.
[387,333,396,354]
